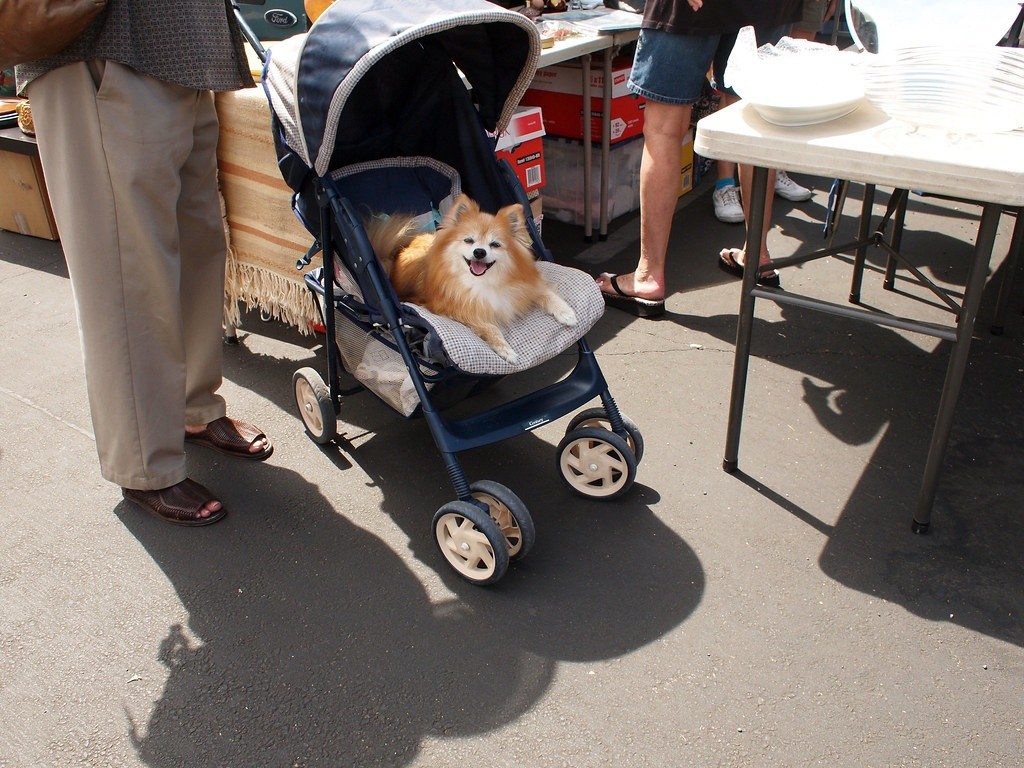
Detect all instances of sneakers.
[774,170,812,202]
[712,187,745,223]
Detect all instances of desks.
[538,7,647,243]
[208,24,613,345]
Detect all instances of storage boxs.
[0,148,59,240]
[488,61,726,249]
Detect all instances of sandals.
[122,478,226,527]
[185,416,273,460]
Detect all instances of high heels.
[719,248,780,287]
[594,272,665,317]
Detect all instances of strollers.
[230,1,648,590]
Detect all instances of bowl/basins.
[751,51,876,127]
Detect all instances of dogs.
[359,194,579,366]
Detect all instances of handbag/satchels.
[0,0,107,72]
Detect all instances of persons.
[595,0,838,320]
[0,0,274,526]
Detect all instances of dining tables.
[693,45,1023,535]
